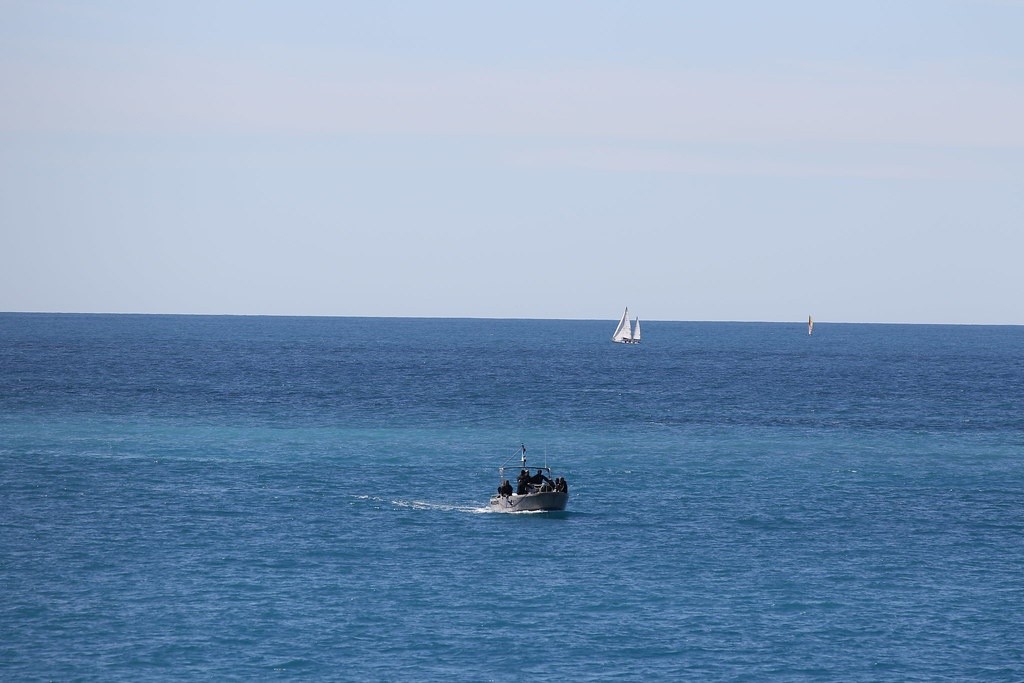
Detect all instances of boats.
[488,441,569,510]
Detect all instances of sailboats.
[611,305,641,344]
[808,313,814,336]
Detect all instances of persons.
[500,469,567,498]
